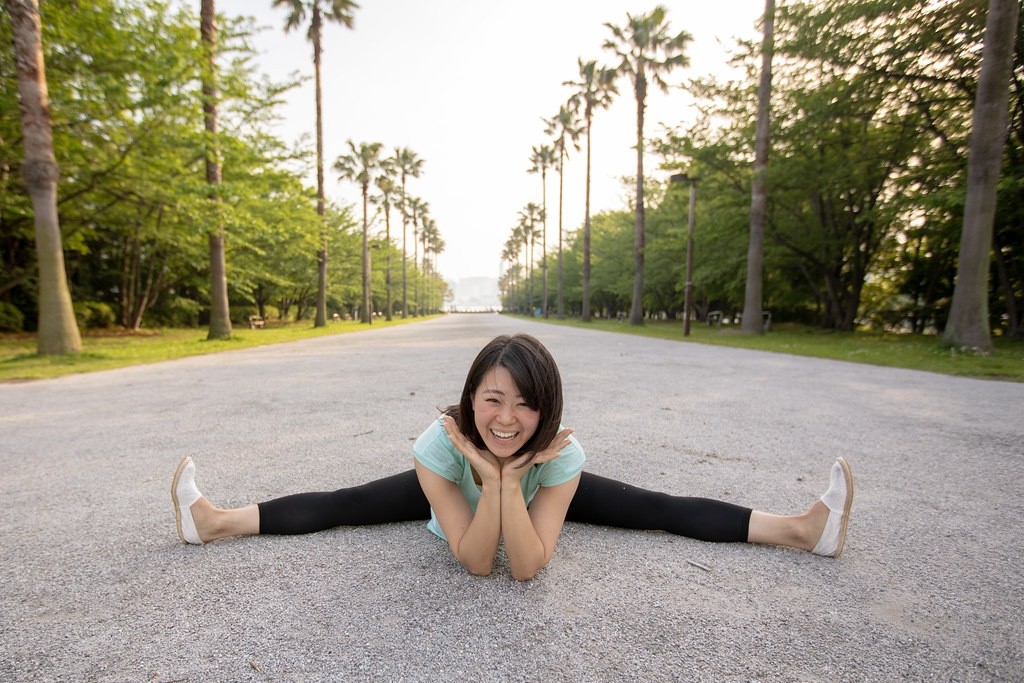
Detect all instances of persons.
[170,334,854,581]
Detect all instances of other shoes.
[173,455,204,545]
[810,457,854,558]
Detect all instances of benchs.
[332,311,403,323]
[248,315,266,329]
